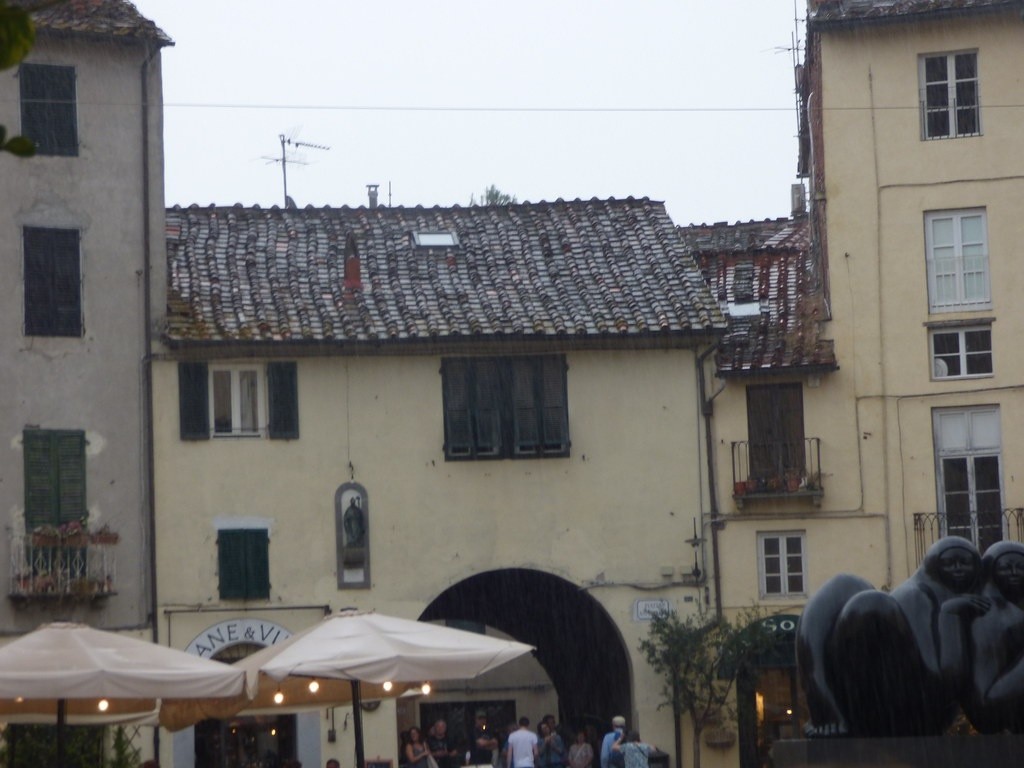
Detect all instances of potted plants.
[15,570,115,594]
[27,517,118,547]
[747,475,760,492]
[784,467,805,492]
[766,464,783,490]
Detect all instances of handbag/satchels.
[424,742,439,768]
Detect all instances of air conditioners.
[791,183,806,214]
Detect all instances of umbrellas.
[232,608,537,768]
[0,622,245,768]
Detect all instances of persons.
[344,497,364,548]
[797,536,1024,737]
[407,712,656,768]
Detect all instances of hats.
[613,716,625,726]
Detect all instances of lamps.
[272,681,285,705]
